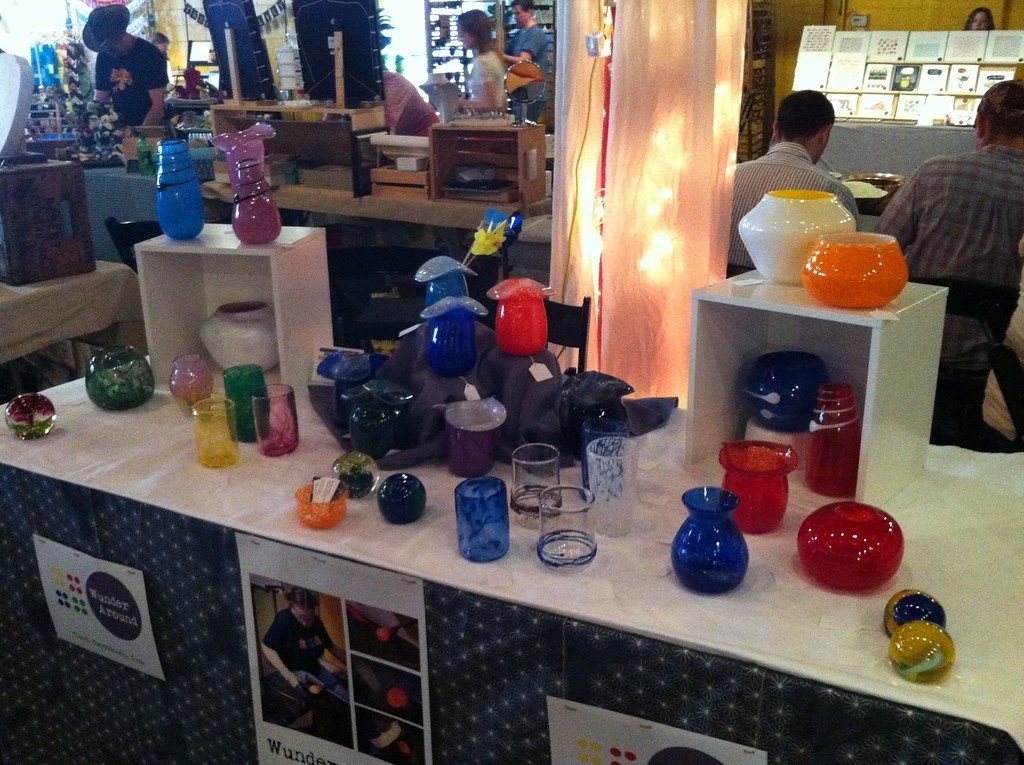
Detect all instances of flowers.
[463,220,507,267]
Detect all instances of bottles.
[210,122,276,191]
[136,134,153,176]
[232,158,282,245]
[155,140,205,240]
[316,255,555,475]
[170,352,213,417]
[669,485,748,595]
[801,230,909,310]
[805,381,860,498]
[198,301,279,372]
[85,345,154,411]
[742,350,830,433]
[798,501,904,592]
[738,189,856,286]
[718,439,798,534]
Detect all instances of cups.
[539,484,597,571]
[190,364,300,467]
[510,443,562,527]
[455,477,510,563]
[295,484,350,528]
[581,418,630,488]
[586,437,639,537]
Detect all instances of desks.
[0,106,1024,761]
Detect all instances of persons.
[962,7,995,32]
[863,80,1024,450]
[382,71,440,137]
[457,10,506,114]
[83,4,169,137]
[725,90,861,280]
[503,0,548,124]
[149,32,170,71]
[261,586,347,715]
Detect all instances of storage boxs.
[0,159,98,285]
[428,125,545,204]
[302,166,353,191]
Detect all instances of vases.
[807,384,863,497]
[671,486,749,595]
[199,300,278,372]
[155,136,204,239]
[738,186,856,285]
[803,232,909,308]
[719,439,799,534]
[210,122,281,243]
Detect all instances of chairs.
[331,242,502,355]
[544,297,591,374]
[104,217,164,274]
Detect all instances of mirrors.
[504,60,546,127]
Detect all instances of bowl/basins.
[844,172,906,214]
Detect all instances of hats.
[84,4,129,54]
